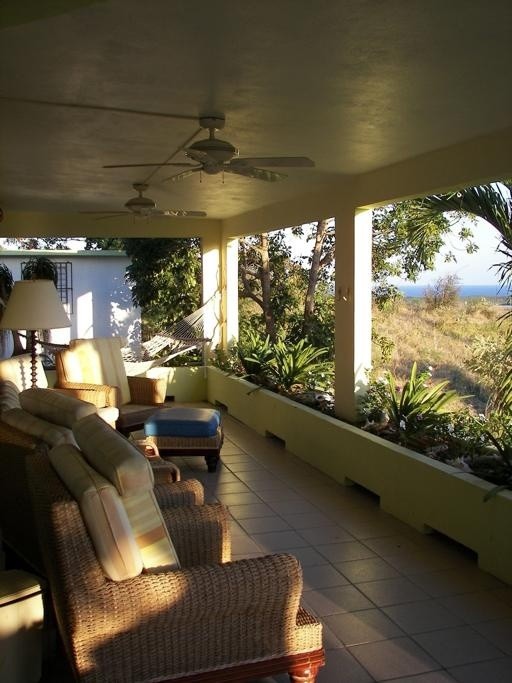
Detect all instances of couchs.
[2,354,119,429]
[26,445,324,683]
[55,338,165,433]
[2,379,160,455]
[2,407,208,576]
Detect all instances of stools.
[144,404,224,474]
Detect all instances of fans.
[78,117,314,222]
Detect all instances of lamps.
[0,279,72,387]
